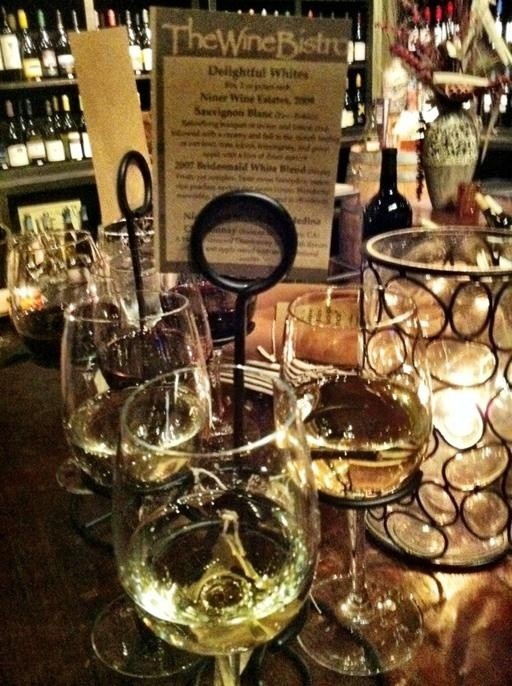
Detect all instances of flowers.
[373,0,512,200]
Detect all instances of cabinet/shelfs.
[1,0,374,287]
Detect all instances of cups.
[6,227,105,361]
[354,224,511,571]
[455,183,479,226]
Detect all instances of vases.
[419,107,481,212]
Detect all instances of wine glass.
[280,284,432,677]
[114,361,319,685]
[56,214,257,678]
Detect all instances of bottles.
[404,1,512,128]
[472,192,511,263]
[1,97,94,170]
[25,206,95,271]
[224,5,368,126]
[0,7,152,84]
